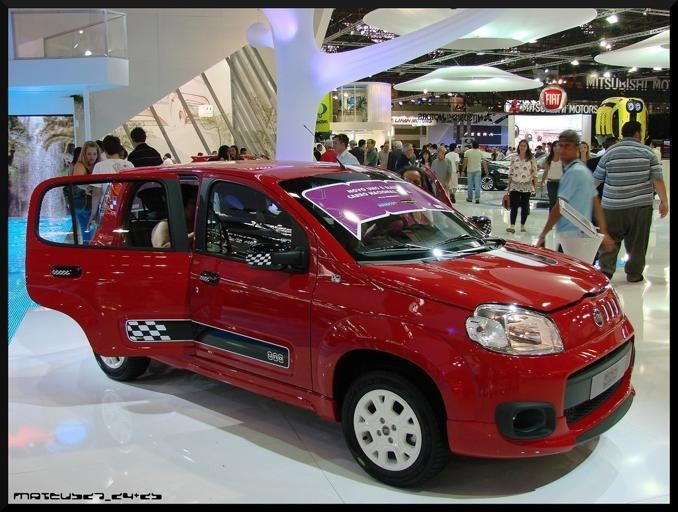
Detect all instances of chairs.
[131,186,170,248]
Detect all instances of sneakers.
[466,199,472,202]
[476,201,479,203]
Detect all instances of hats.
[559,130,579,143]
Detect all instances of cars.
[430,152,510,191]
[530,151,606,170]
[23,154,639,488]
[503,150,539,161]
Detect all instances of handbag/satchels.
[502,193,510,209]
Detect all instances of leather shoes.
[521,230,526,232]
[506,229,515,233]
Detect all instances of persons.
[70,120,670,283]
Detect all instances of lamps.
[393,65,543,94]
[594,28,670,70]
[363,8,597,53]
